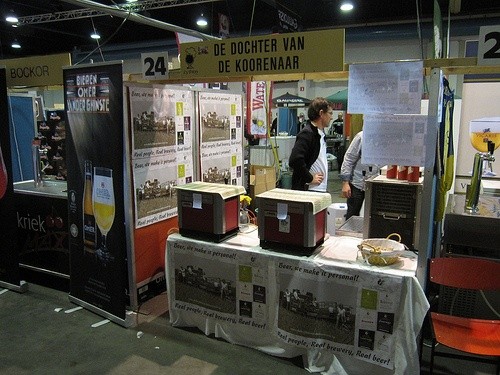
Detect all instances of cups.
[407,167,419,182]
[398,166,407,179]
[387,165,397,179]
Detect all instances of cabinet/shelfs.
[37,110,66,181]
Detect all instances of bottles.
[82,161,97,256]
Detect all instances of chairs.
[418,257,500,375]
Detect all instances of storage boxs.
[173,180,246,243]
[255,188,332,257]
[362,173,424,251]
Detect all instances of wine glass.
[469,117,500,177]
[92,167,115,258]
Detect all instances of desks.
[164,223,431,375]
[439,194,500,314]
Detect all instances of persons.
[329,111,343,133]
[270,113,278,136]
[339,128,367,222]
[289,96,335,192]
[296,112,307,133]
[244,116,260,146]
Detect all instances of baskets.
[356,233,406,267]
